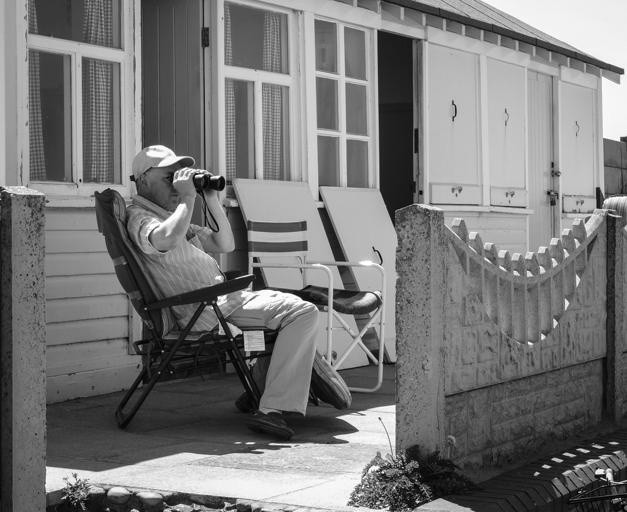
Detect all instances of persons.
[127,143,319,442]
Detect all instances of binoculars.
[169,173,226,191]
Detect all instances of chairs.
[93,188,388,429]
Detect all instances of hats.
[130,144,196,183]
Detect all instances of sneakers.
[247,409,296,441]
[235,391,258,414]
